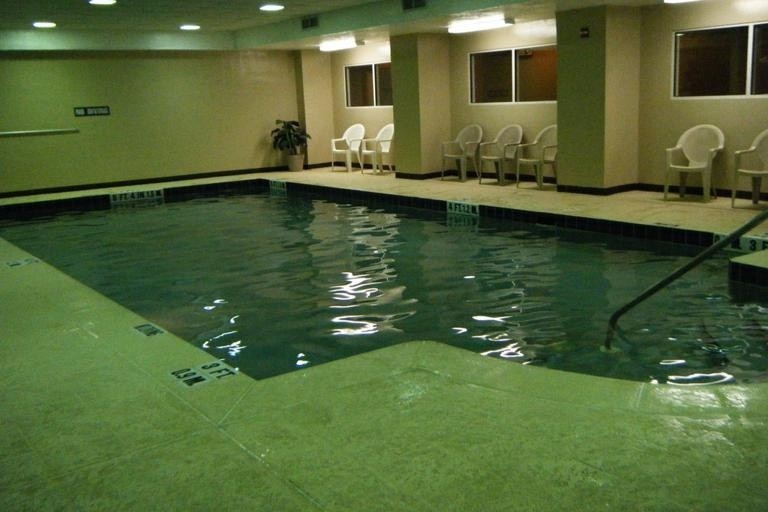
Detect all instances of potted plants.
[270,119,313,172]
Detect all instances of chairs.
[663,122,767,210]
[330,123,557,190]
